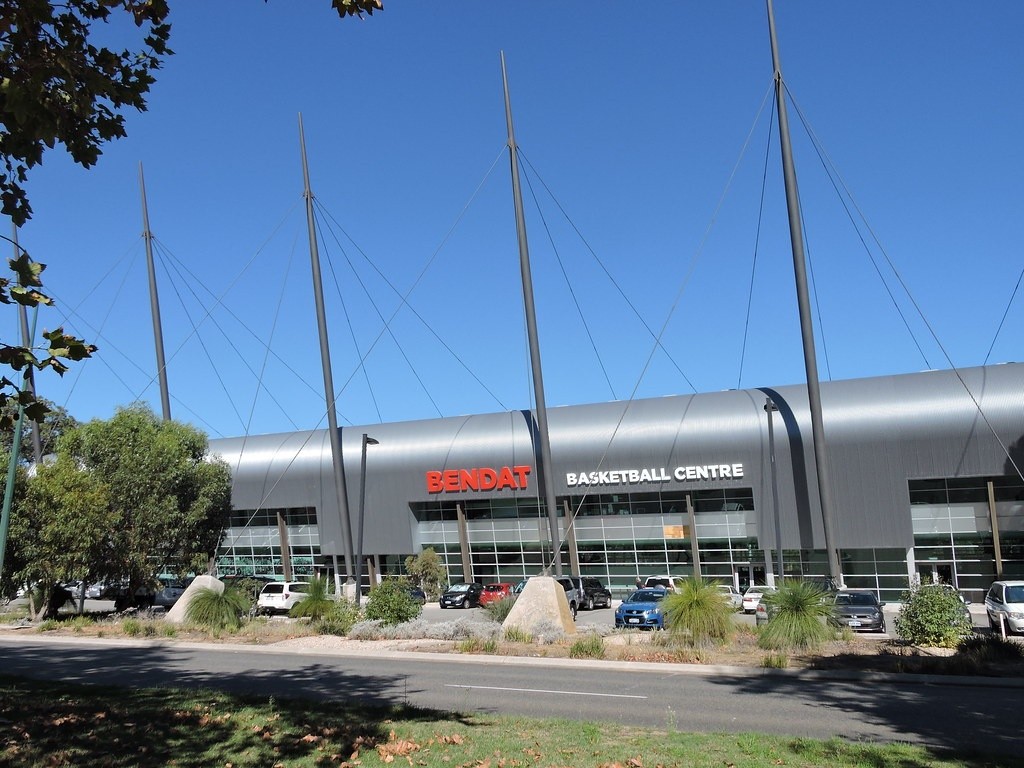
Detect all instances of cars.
[911,583,972,625]
[756,587,792,630]
[409,582,427,607]
[741,585,773,614]
[825,590,886,634]
[705,584,745,613]
[438,582,483,609]
[984,579,1024,635]
[3,572,274,610]
[478,582,516,609]
[614,590,679,634]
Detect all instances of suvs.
[509,575,581,623]
[565,574,614,611]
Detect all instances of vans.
[257,581,314,614]
[643,574,691,601]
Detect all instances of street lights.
[764,397,786,586]
[354,431,380,608]
